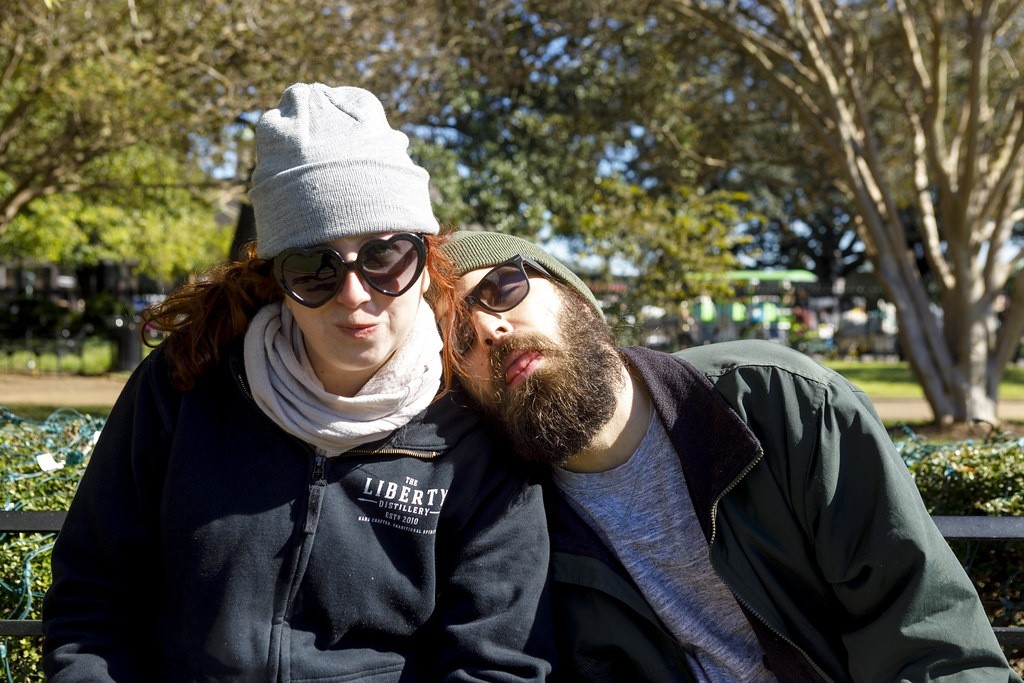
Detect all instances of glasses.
[434,256,554,357]
[257,232,427,310]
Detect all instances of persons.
[424,229,1024,683]
[42,81,550,683]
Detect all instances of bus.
[572,268,822,355]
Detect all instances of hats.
[429,226,607,324]
[246,81,441,260]
